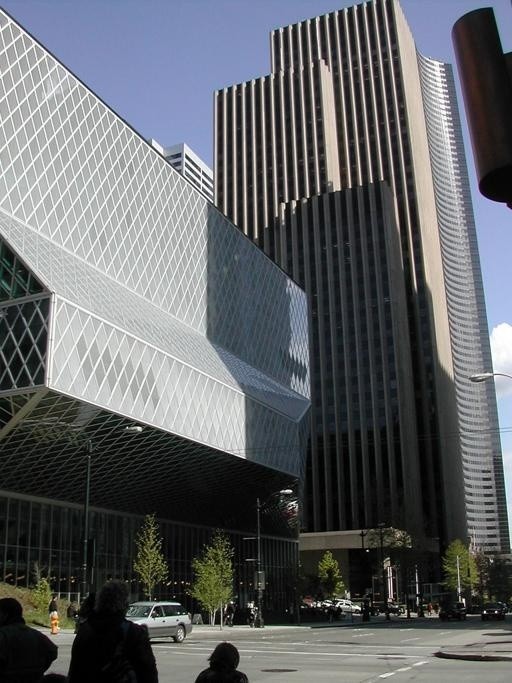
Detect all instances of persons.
[223,597,259,629]
[66,578,161,683]
[0,596,58,683]
[48,594,59,635]
[194,641,251,683]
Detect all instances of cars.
[124,597,195,644]
[312,598,406,616]
[438,601,468,621]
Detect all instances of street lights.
[454,550,475,602]
[360,520,388,621]
[253,487,297,629]
[74,424,145,633]
[466,370,512,384]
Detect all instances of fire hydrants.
[49,609,59,634]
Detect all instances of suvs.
[480,601,506,619]
